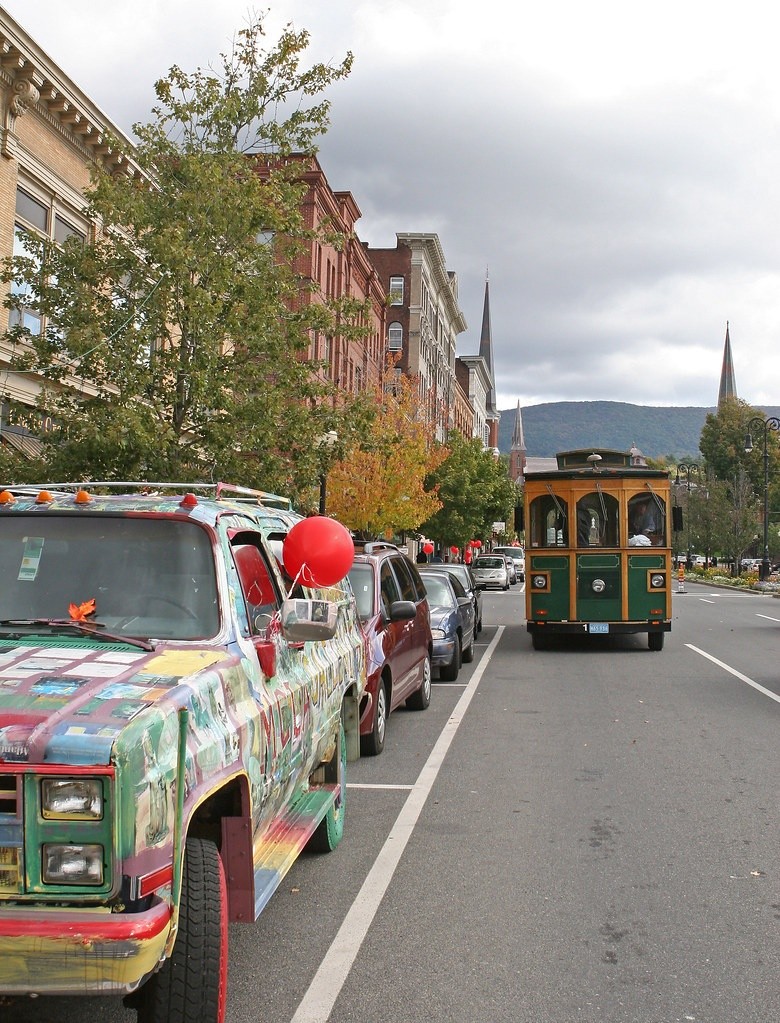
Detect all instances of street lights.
[313,431,338,515]
[744,417,780,580]
[674,463,703,570]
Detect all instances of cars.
[468,553,517,592]
[671,555,713,568]
[413,562,483,642]
[416,569,475,682]
[740,559,772,574]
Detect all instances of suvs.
[0,481,372,1022]
[347,540,434,757]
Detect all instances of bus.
[514,449,684,652]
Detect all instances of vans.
[490,546,525,582]
